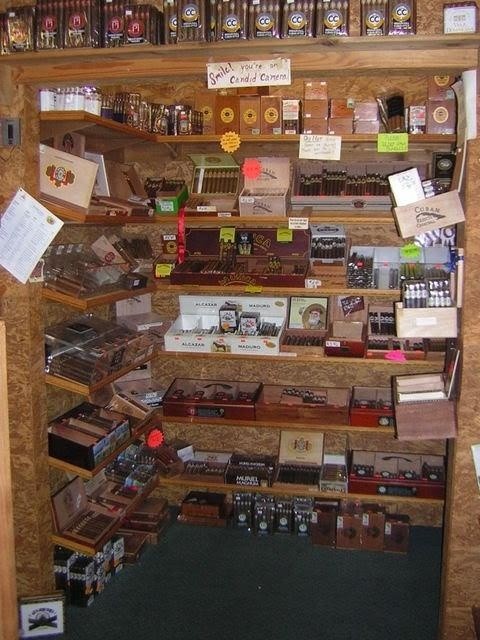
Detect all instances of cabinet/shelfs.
[154,131,461,506]
[39,110,156,607]
